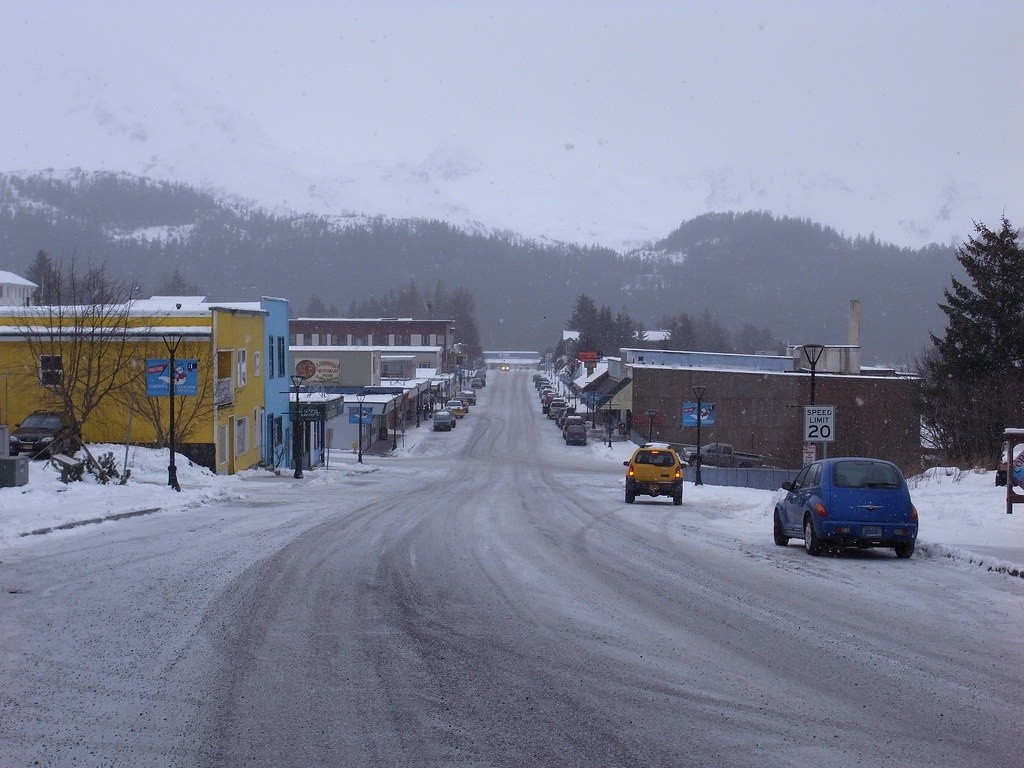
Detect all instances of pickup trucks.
[681,442,764,470]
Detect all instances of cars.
[533,374,582,439]
[446,368,486,418]
[433,412,455,431]
[9,410,83,458]
[774,457,919,561]
[563,425,587,446]
[624,445,685,508]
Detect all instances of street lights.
[803,344,827,462]
[356,393,367,467]
[646,409,656,443]
[163,330,183,489]
[607,395,614,447]
[416,383,421,426]
[592,382,596,429]
[291,375,308,478]
[585,381,590,419]
[544,354,577,409]
[391,392,398,453]
[692,386,706,485]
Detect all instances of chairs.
[640,455,649,465]
[663,457,671,467]
